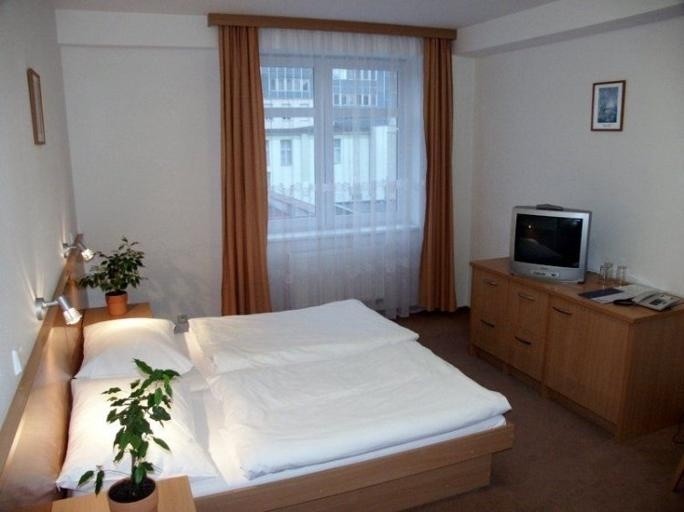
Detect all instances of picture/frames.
[28,67,46,145]
[591,80,626,133]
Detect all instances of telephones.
[632,291,681,311]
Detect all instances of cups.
[616,265,627,286]
[600,264,613,290]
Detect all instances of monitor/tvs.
[510,205,591,283]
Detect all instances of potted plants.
[74,358,181,512]
[73,236,149,316]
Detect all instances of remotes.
[536,204,563,210]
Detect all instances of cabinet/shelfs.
[468,253,684,438]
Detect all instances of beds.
[1,297,515,511]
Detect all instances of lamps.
[35,295,84,326]
[63,239,95,263]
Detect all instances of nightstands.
[52,475,198,512]
[81,302,153,327]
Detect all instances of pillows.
[74,317,195,379]
[53,378,221,486]
